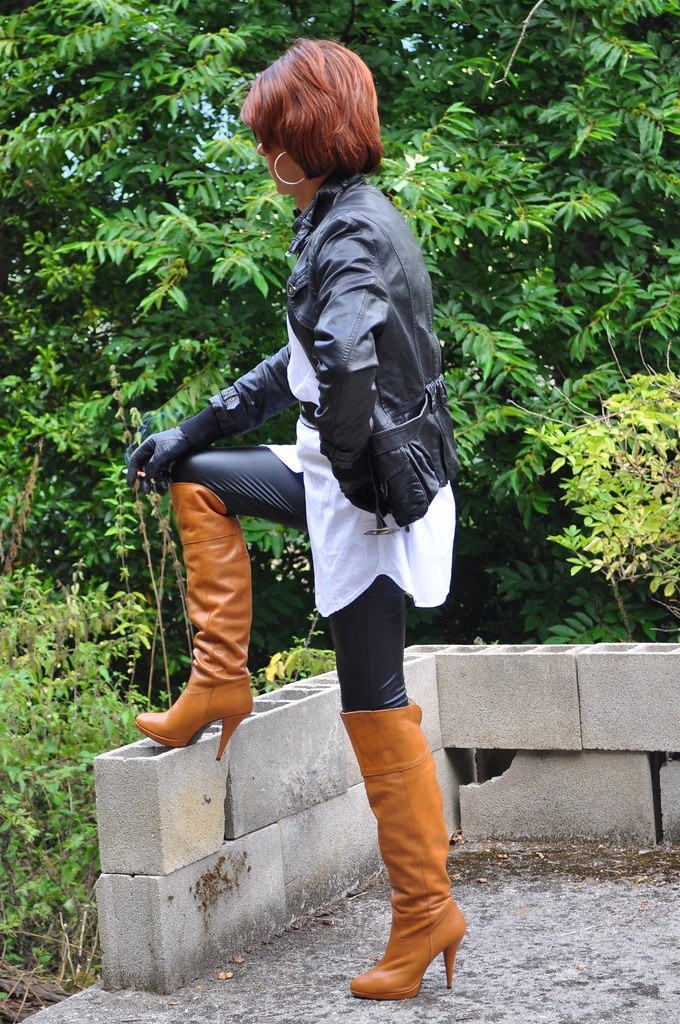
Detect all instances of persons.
[126,47,467,1001]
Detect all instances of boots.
[340,695,466,999]
[134,481,253,762]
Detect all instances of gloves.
[332,462,387,521]
[126,425,189,496]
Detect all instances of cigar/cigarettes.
[121,469,145,478]
